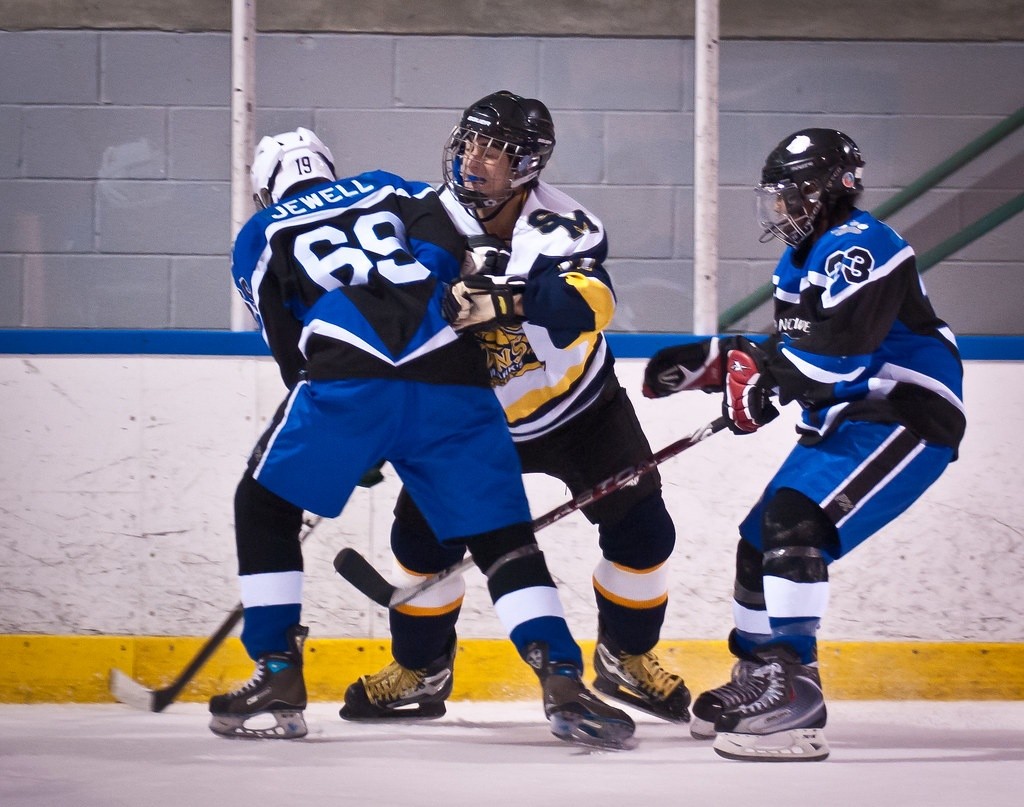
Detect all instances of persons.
[206,127,639,752]
[340,91,693,724]
[642,127,966,763]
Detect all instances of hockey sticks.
[107,503,325,715]
[333,412,733,611]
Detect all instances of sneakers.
[339,632,457,722]
[592,629,691,724]
[713,642,829,761]
[521,640,635,752]
[689,637,770,740]
[209,623,309,740]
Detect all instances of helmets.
[759,127,864,215]
[461,90,555,190]
[250,127,336,208]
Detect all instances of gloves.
[441,275,526,334]
[642,336,723,400]
[723,335,780,435]
